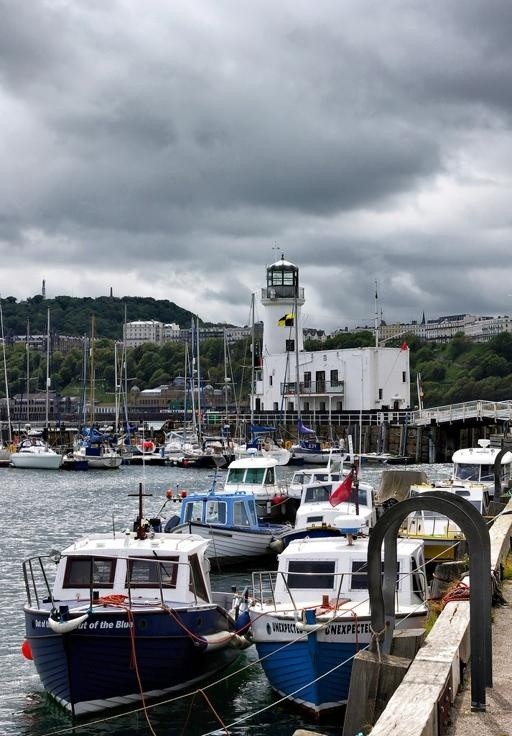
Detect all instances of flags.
[401,342,407,350]
[277,313,294,326]
[329,471,353,507]
